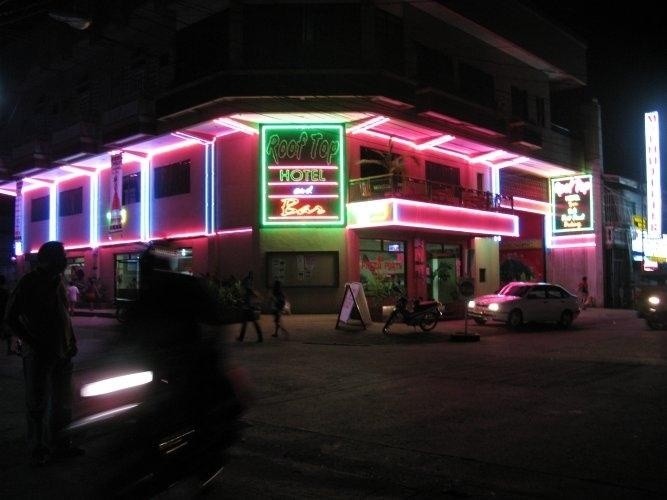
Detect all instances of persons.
[576,276,588,312]
[66,280,80,316]
[82,278,101,312]
[4,241,88,465]
[114,239,235,440]
[234,286,264,344]
[270,280,289,338]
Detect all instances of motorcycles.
[634,270,667,331]
[382,288,448,335]
[111,297,135,323]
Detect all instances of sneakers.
[31,449,46,466]
[60,445,86,458]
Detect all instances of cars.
[467,282,581,328]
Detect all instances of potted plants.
[353,135,423,198]
